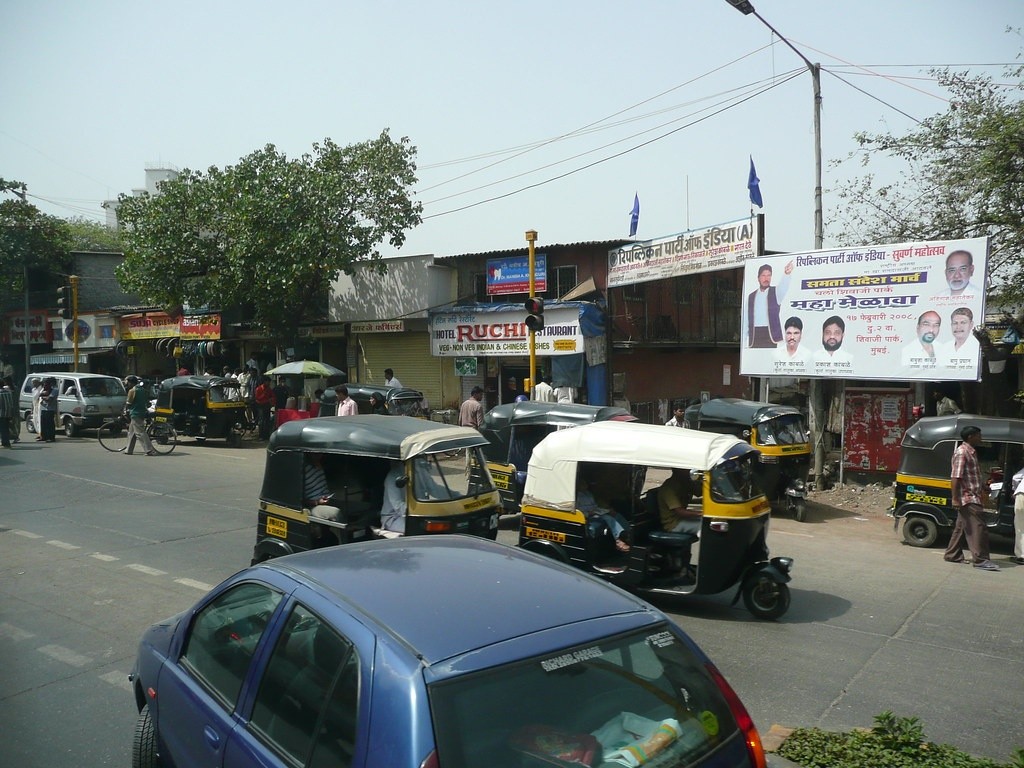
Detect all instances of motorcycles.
[886,413,1024,547]
[316,383,428,420]
[250,414,505,567]
[684,397,812,522]
[513,421,794,619]
[470,402,639,515]
[153,374,249,448]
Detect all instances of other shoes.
[145,450,156,456]
[46,439,55,442]
[13,439,20,443]
[36,438,45,441]
[973,560,999,570]
[1015,558,1024,564]
[616,542,630,552]
[945,558,970,564]
[258,437,266,441]
[121,450,133,454]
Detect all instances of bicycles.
[96,401,178,455]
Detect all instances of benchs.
[576,480,632,537]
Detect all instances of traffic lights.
[57,286,72,319]
[525,297,545,331]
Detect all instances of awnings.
[31,348,111,366]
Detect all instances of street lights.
[727,0,823,493]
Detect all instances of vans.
[19,372,128,438]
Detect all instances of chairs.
[364,495,406,542]
[646,487,698,547]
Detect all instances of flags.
[748,158,763,208]
[629,195,639,236]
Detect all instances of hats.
[126,376,137,382]
[31,378,39,381]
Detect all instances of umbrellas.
[263,359,346,394]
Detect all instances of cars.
[133,532,767,768]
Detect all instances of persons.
[380,461,463,532]
[932,385,961,416]
[203,352,291,440]
[334,369,428,416]
[665,396,699,430]
[779,425,810,443]
[577,490,630,551]
[902,308,980,361]
[176,362,191,376]
[743,260,853,376]
[305,452,344,524]
[944,250,979,296]
[122,376,157,455]
[0,353,94,450]
[459,386,483,468]
[657,469,702,540]
[534,376,578,404]
[944,426,1024,570]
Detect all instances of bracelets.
[316,501,317,506]
